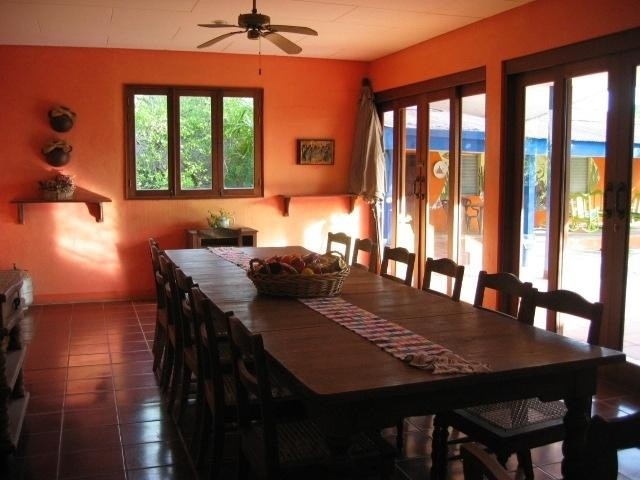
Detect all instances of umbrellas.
[343,77,389,276]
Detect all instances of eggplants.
[257,261,298,274]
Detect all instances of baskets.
[248,251,351,298]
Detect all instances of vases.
[216,216,235,229]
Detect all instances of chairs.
[569,189,640,233]
[441,196,480,231]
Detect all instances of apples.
[301,268,314,275]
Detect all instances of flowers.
[207,207,235,229]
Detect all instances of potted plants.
[535,176,547,228]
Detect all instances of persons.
[301,143,330,163]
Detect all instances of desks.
[464,204,483,230]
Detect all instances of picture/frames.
[297,139,335,165]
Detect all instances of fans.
[196,0,318,54]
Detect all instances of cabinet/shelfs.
[184,227,259,249]
[0,270,30,450]
